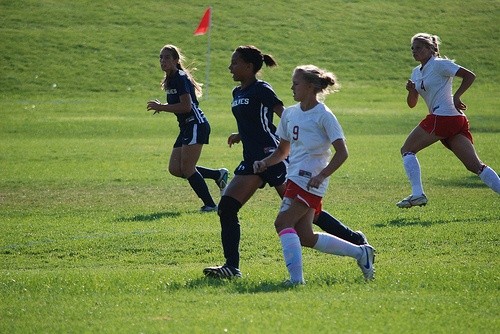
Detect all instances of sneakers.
[396,193,428,209]
[216,168,231,197]
[357,243,376,281]
[202,266,242,280]
[201,204,218,211]
[355,231,368,245]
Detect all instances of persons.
[204,46,368,279]
[146,44,229,213]
[397,33,500,208]
[254,65,375,288]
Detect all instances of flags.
[195,8,210,35]
